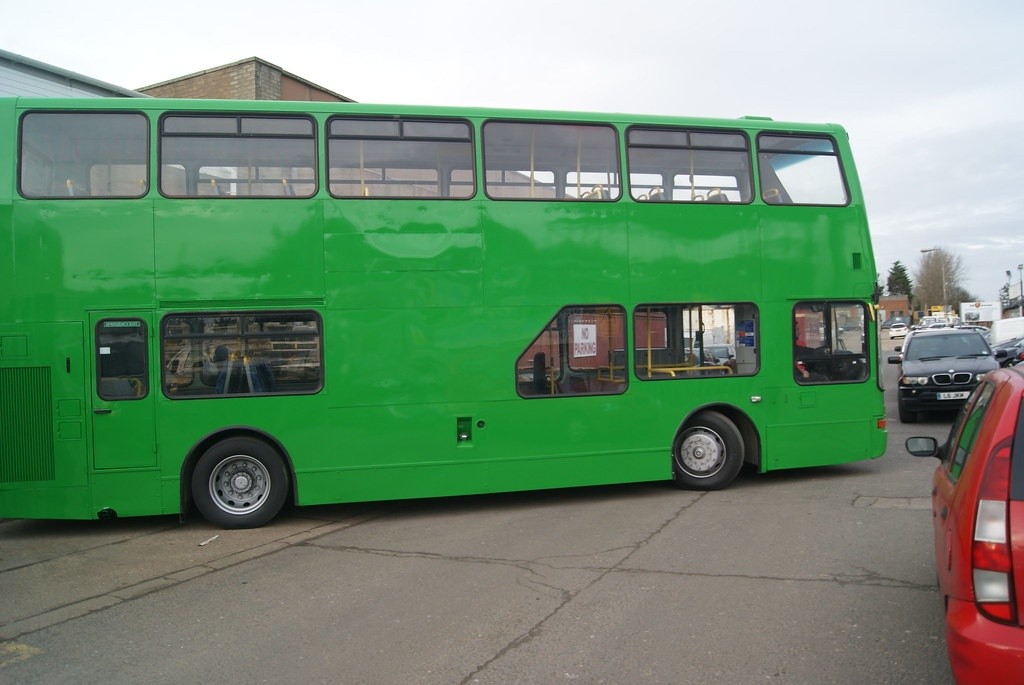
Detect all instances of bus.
[1,92,887,530]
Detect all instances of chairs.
[648,187,665,200]
[211,179,221,195]
[282,179,291,195]
[139,179,145,194]
[706,187,730,203]
[364,187,369,197]
[762,188,784,205]
[581,185,611,200]
[691,194,705,202]
[66,180,83,196]
[638,194,648,201]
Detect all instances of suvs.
[886,327,1003,425]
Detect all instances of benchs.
[531,352,549,396]
[216,356,272,392]
[608,346,680,373]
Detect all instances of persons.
[794,320,829,382]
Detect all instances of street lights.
[1017,263,1024,309]
[920,248,949,327]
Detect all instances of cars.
[912,315,1024,369]
[887,323,909,340]
[904,358,1024,685]
